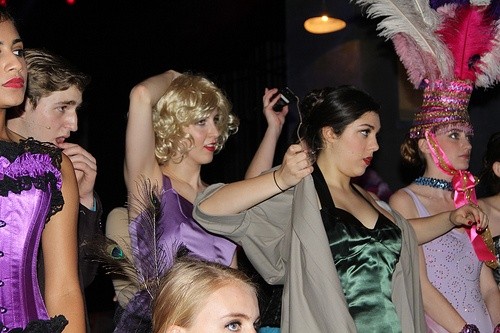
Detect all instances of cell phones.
[272,87,296,110]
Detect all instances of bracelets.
[459,324,480,333]
[272,169,286,192]
[494,324,500,333]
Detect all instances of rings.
[472,204,480,208]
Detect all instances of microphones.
[21,117,50,129]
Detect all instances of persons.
[6,48,104,333]
[475,132,500,293]
[190,84,428,333]
[0,9,88,333]
[388,65,500,333]
[111,70,238,333]
[149,256,262,333]
[107,207,154,327]
[244,86,289,180]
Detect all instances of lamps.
[303,0,348,35]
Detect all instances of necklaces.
[414,176,454,191]
